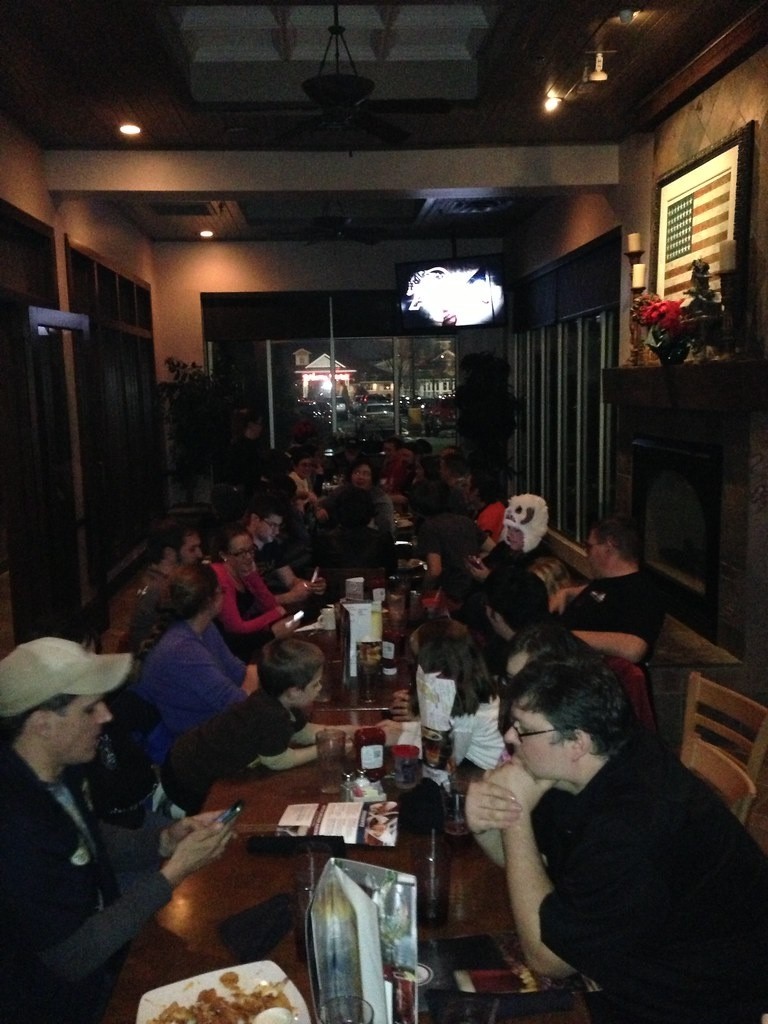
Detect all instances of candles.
[632,263,648,288]
[720,239,737,269]
[627,232,640,252]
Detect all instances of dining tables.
[102,508,594,1024]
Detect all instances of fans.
[281,196,390,245]
[264,5,453,146]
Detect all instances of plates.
[135,961,312,1024]
[399,559,424,568]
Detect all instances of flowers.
[641,299,687,352]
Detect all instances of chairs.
[679,672,768,828]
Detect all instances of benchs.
[542,526,746,747]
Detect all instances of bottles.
[382,632,400,674]
[340,770,357,802]
[368,577,386,608]
[354,768,370,790]
[353,726,386,787]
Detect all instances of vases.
[652,330,692,364]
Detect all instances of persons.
[70,407,671,830]
[464,646,768,1024]
[162,635,403,817]
[0,635,239,1024]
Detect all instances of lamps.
[588,53,608,80]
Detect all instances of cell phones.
[311,567,319,583]
[285,610,304,627]
[467,556,482,570]
[216,799,245,825]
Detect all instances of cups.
[318,608,335,630]
[289,853,329,921]
[409,826,451,930]
[391,743,419,789]
[315,729,345,794]
[440,779,468,834]
[357,658,380,703]
[420,722,453,770]
[318,995,374,1024]
[421,590,446,616]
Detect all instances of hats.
[0,637,131,717]
[503,494,549,554]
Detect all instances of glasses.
[260,519,285,530]
[507,720,589,743]
[230,544,257,560]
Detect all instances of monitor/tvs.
[396,251,509,335]
